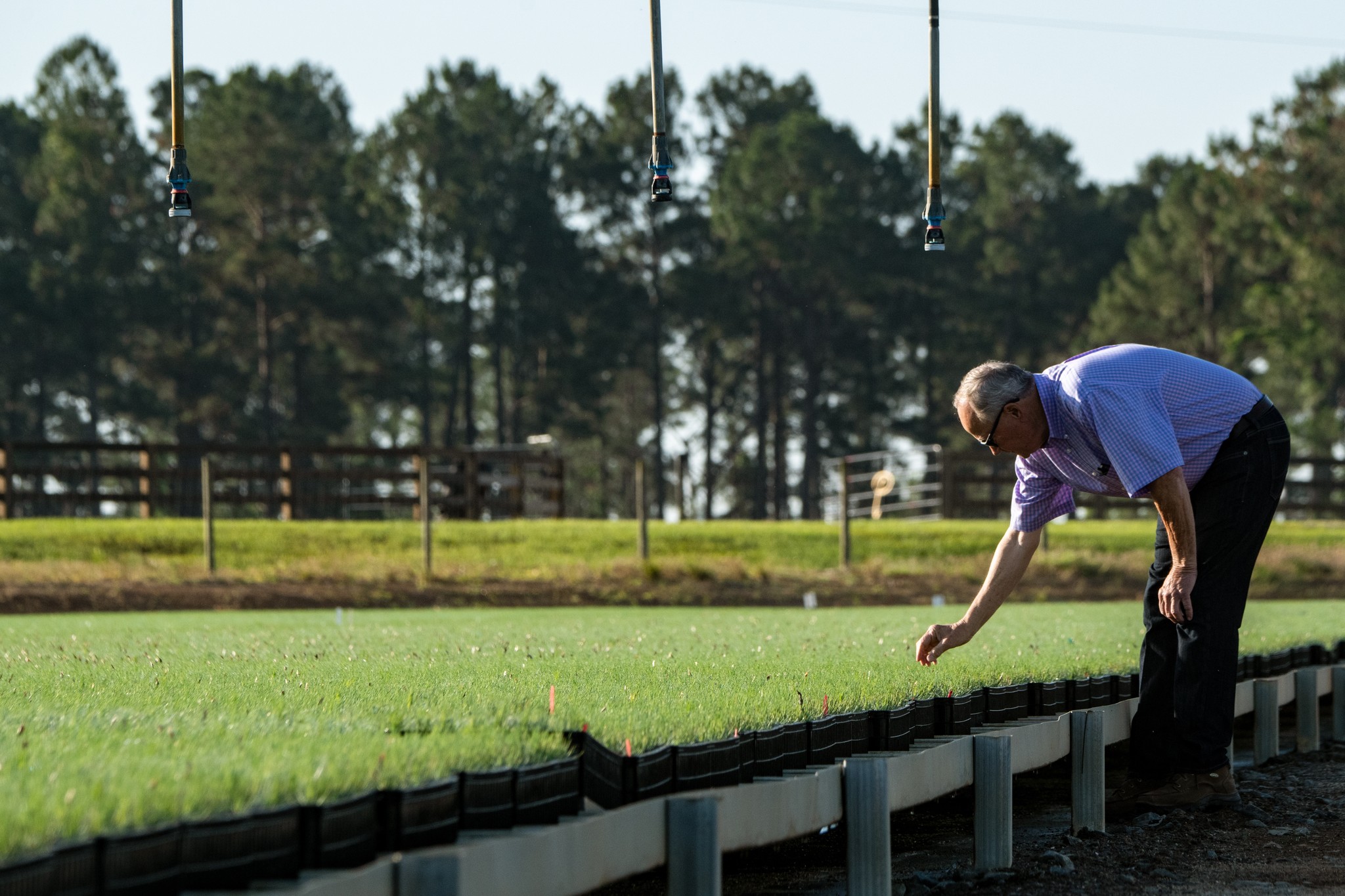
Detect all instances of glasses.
[982,397,1021,446]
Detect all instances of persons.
[915,339,1292,811]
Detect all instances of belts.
[1238,394,1273,428]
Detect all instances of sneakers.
[1135,764,1241,810]
[1106,772,1154,808]
